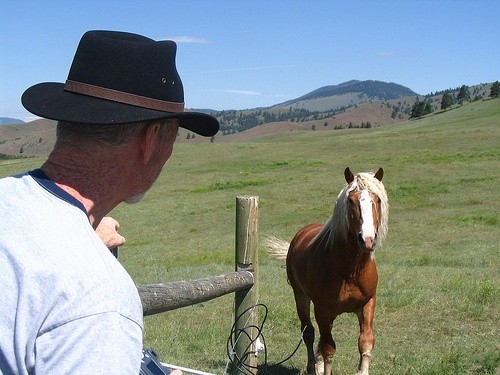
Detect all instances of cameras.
[139,347,170,375]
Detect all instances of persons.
[0,30,220,375]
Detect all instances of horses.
[263,166,389,375]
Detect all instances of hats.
[21,30,219,137]
